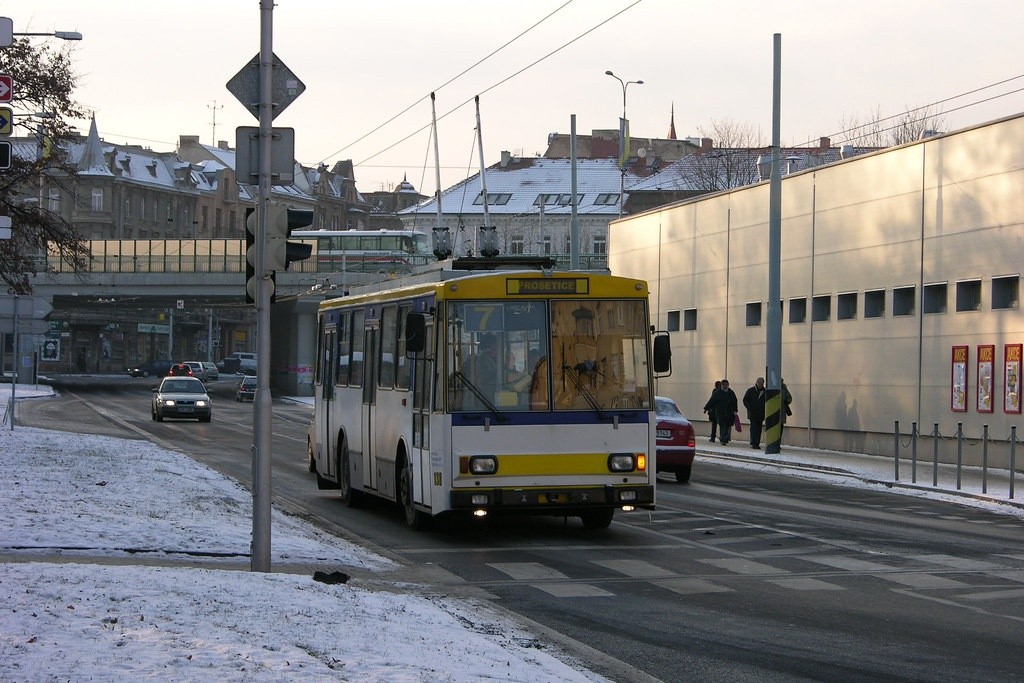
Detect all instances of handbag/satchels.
[735,414,742,432]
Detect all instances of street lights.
[10,252,48,429]
[604,70,644,219]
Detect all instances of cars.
[168,360,220,383]
[151,375,214,422]
[306,410,316,473]
[235,376,257,403]
[654,395,696,483]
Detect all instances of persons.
[703,380,738,445]
[464,331,623,407]
[743,377,765,449]
[779,378,792,449]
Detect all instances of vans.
[217,352,258,376]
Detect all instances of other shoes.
[750,444,756,450]
[709,439,715,443]
[756,444,761,449]
[721,442,727,446]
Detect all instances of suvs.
[127,359,181,378]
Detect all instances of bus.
[312,93,674,532]
[289,227,432,275]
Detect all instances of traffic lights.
[260,202,314,276]
